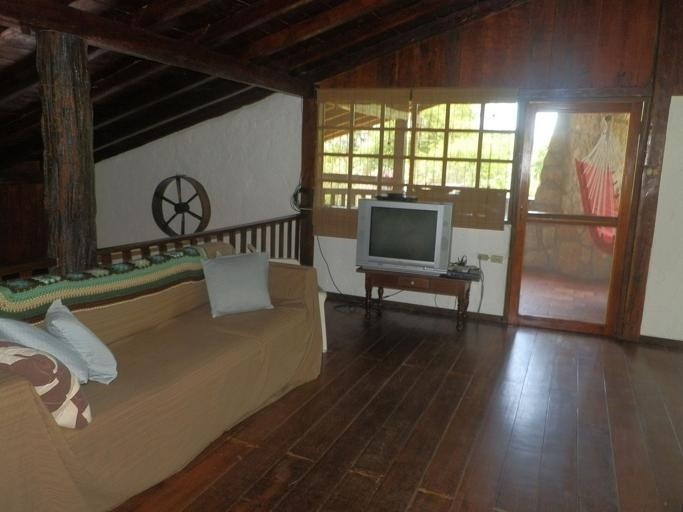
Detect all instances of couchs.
[0,240,323,511]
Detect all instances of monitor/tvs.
[355,198,454,277]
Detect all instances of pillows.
[199,251,276,319]
[0,297,120,429]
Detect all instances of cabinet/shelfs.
[356,266,480,332]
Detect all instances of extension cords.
[453,263,469,273]
